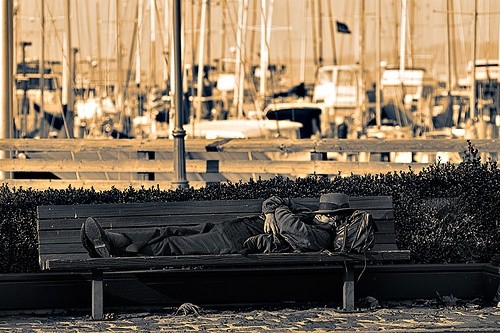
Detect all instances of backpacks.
[332,210,380,283]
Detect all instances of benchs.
[37,195,411,320]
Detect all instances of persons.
[79,192,355,258]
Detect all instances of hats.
[302,193,357,213]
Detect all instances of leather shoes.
[85,217,112,257]
[80,223,97,258]
